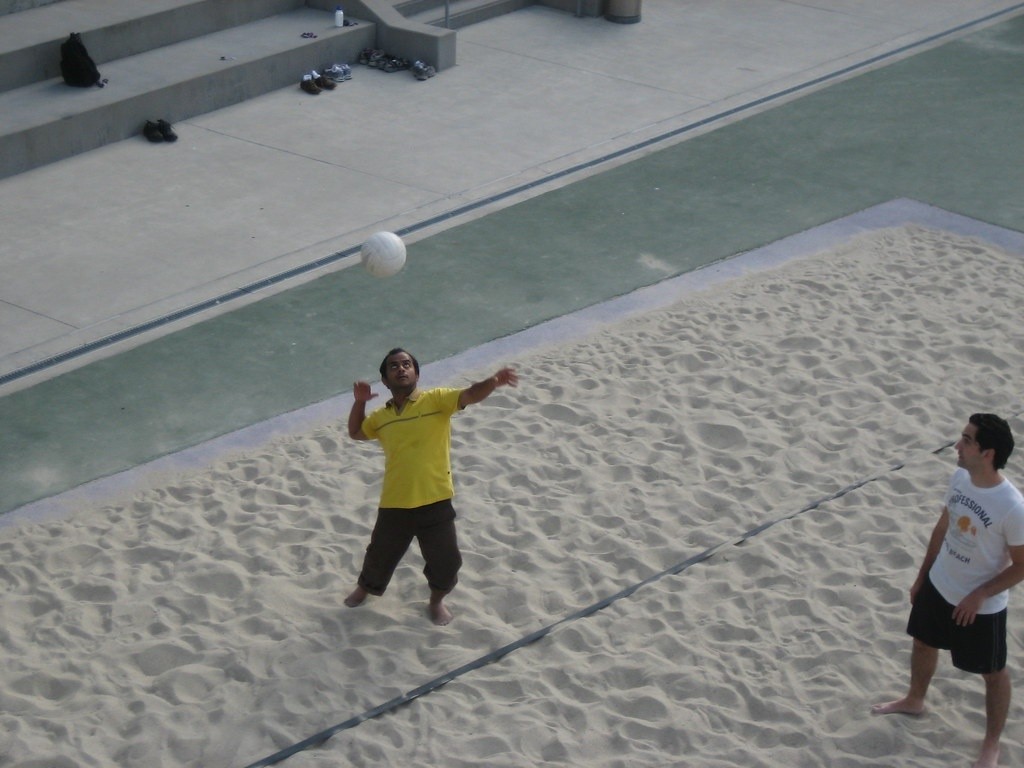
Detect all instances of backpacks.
[60,31,101,88]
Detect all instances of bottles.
[335,6,343,27]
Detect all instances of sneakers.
[368,50,385,66]
[413,60,425,77]
[335,63,353,79]
[417,65,435,80]
[360,49,374,65]
[324,65,344,81]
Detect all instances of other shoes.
[378,56,394,70]
[143,119,164,142]
[300,76,321,94]
[159,119,178,143]
[383,58,411,72]
[312,73,337,89]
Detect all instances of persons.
[871,413,1023,768]
[343,348,519,625]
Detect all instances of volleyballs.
[360,230,409,278]
